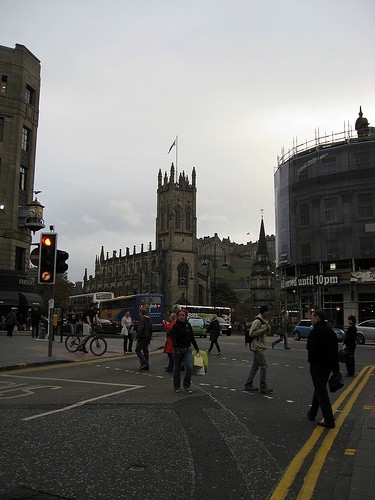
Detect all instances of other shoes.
[78,344,88,353]
[260,387,273,392]
[272,342,275,350]
[307,411,315,421]
[285,348,291,350]
[175,389,181,393]
[244,384,258,391]
[138,362,148,369]
[317,415,335,429]
[185,387,192,393]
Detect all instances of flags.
[167,141,175,154]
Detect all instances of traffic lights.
[56,249,69,273]
[38,231,58,284]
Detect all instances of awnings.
[0,291,45,308]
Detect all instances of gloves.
[194,347,200,352]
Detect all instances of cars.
[292,318,346,342]
[52,316,120,336]
[204,316,232,336]
[354,319,375,345]
[186,317,207,338]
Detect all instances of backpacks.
[244,317,266,343]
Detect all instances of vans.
[277,310,300,333]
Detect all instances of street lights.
[201,242,229,307]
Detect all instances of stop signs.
[30,248,38,266]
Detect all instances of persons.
[167,308,200,394]
[30,307,41,339]
[162,313,176,372]
[245,304,274,393]
[205,314,223,357]
[5,310,17,337]
[272,310,292,350]
[306,310,341,429]
[77,303,101,352]
[134,308,151,370]
[121,309,135,356]
[343,314,358,377]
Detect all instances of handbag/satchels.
[83,323,92,336]
[339,350,350,364]
[121,325,128,335]
[329,375,344,392]
[191,350,209,376]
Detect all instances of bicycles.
[64,324,107,356]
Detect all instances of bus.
[66,292,114,319]
[97,292,165,332]
[168,304,232,326]
